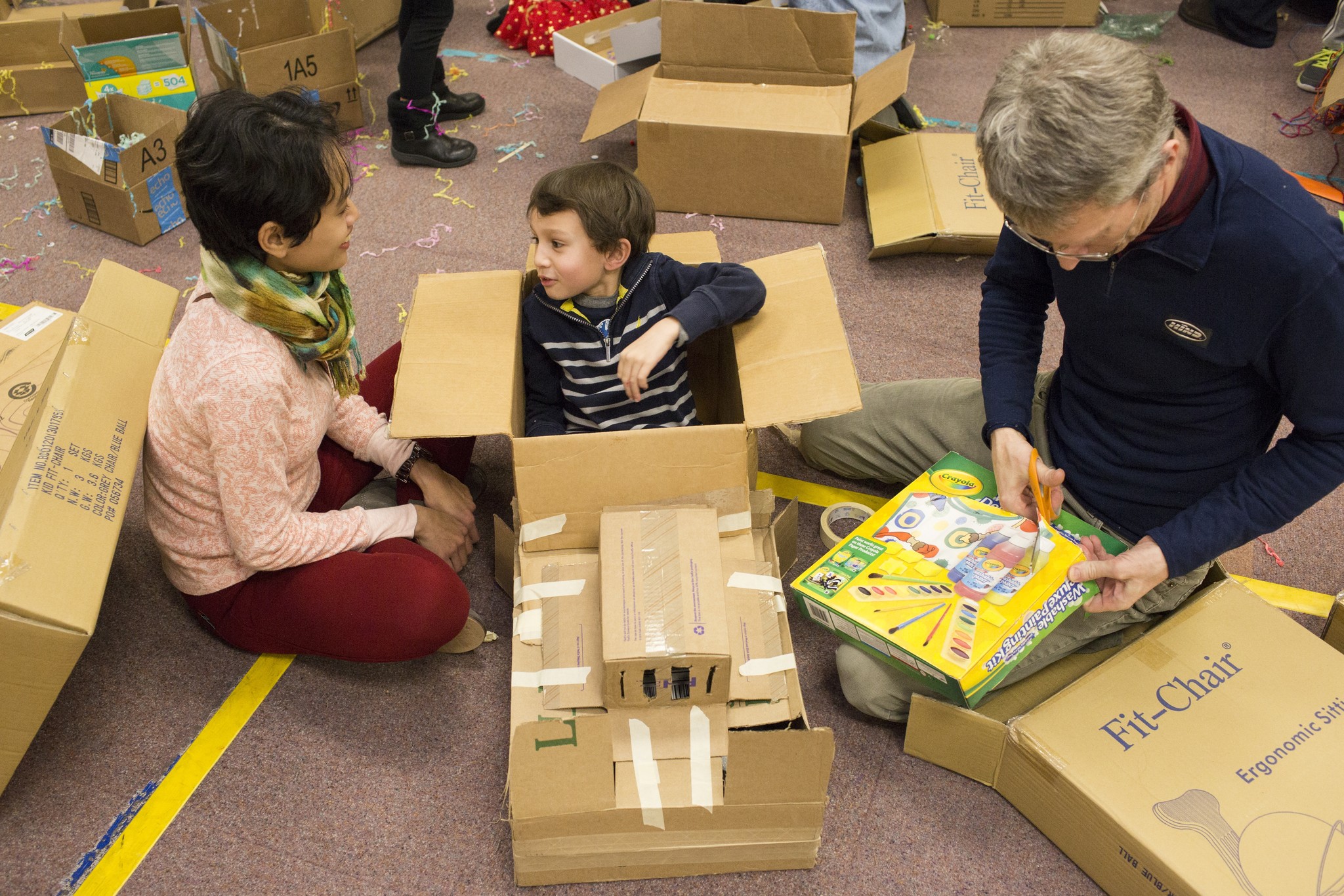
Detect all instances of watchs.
[394,444,435,484]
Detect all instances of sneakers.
[1294,44,1340,91]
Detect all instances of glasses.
[1002,180,1150,263]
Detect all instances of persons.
[793,31,1344,720]
[1297,0,1344,93]
[522,161,767,442]
[143,90,487,658]
[388,0,484,167]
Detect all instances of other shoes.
[1178,1,1224,35]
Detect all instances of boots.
[340,463,487,510]
[438,610,486,654]
[387,90,477,167]
[430,58,486,122]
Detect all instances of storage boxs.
[0,0,1344,896]
